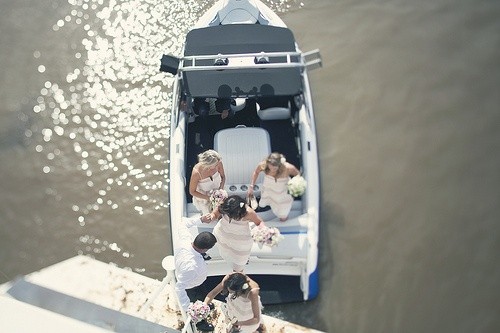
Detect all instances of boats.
[160,0,322,305]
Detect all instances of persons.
[200,195,266,273]
[247,153,300,222]
[189,150,225,215]
[174,231,226,330]
[203,271,263,333]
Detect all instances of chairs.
[255,100,291,120]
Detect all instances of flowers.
[281,158,286,163]
[243,283,249,289]
[286,175,307,197]
[240,202,244,208]
[251,225,282,248]
[209,189,227,207]
[188,300,210,322]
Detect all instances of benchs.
[187,204,305,231]
